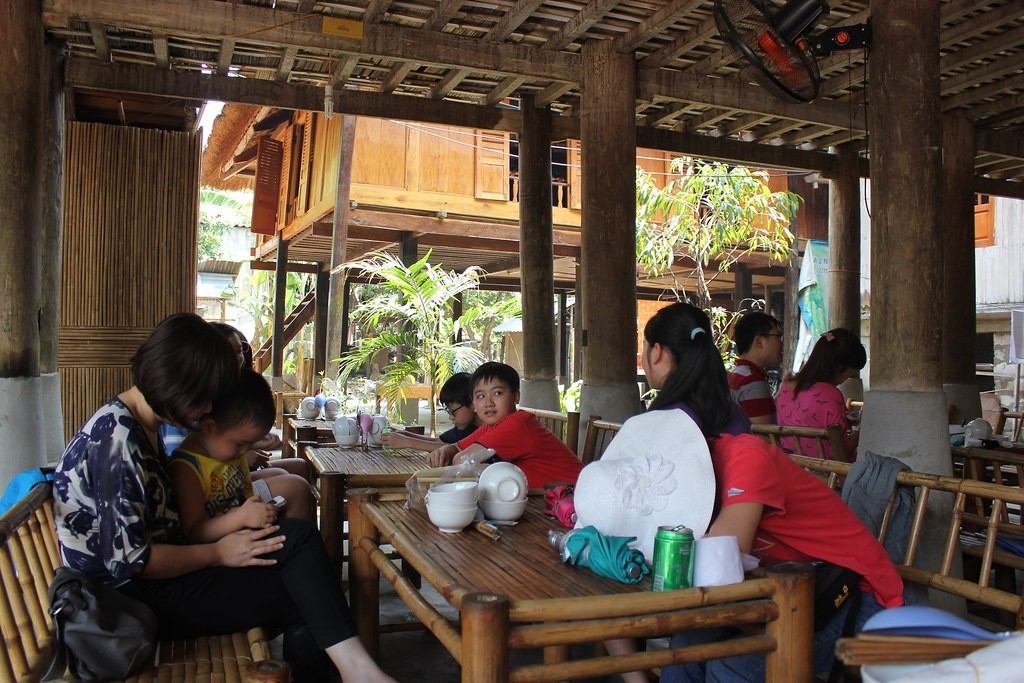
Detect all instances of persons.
[603,302,751,683]
[727,312,784,453]
[776,327,867,462]
[574,408,899,683]
[53,312,399,683]
[427,361,589,491]
[236,331,307,482]
[157,322,318,533]
[167,369,285,555]
[381,372,509,465]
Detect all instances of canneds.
[650,525,696,591]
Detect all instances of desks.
[349,481,814,683]
[281,412,425,460]
[295,442,454,581]
[376,382,440,424]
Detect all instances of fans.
[713,0,873,105]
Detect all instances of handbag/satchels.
[814,562,857,630]
[48,567,155,683]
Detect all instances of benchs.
[0,406,1023,683]
[272,356,314,429]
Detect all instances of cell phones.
[267,496,286,507]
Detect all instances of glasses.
[753,333,783,343]
[445,405,465,416]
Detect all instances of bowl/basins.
[301,398,388,448]
[426,461,529,528]
[964,418,995,442]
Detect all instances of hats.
[574,407,716,568]
[0,467,55,517]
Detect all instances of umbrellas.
[548,525,649,585]
[544,486,578,527]
